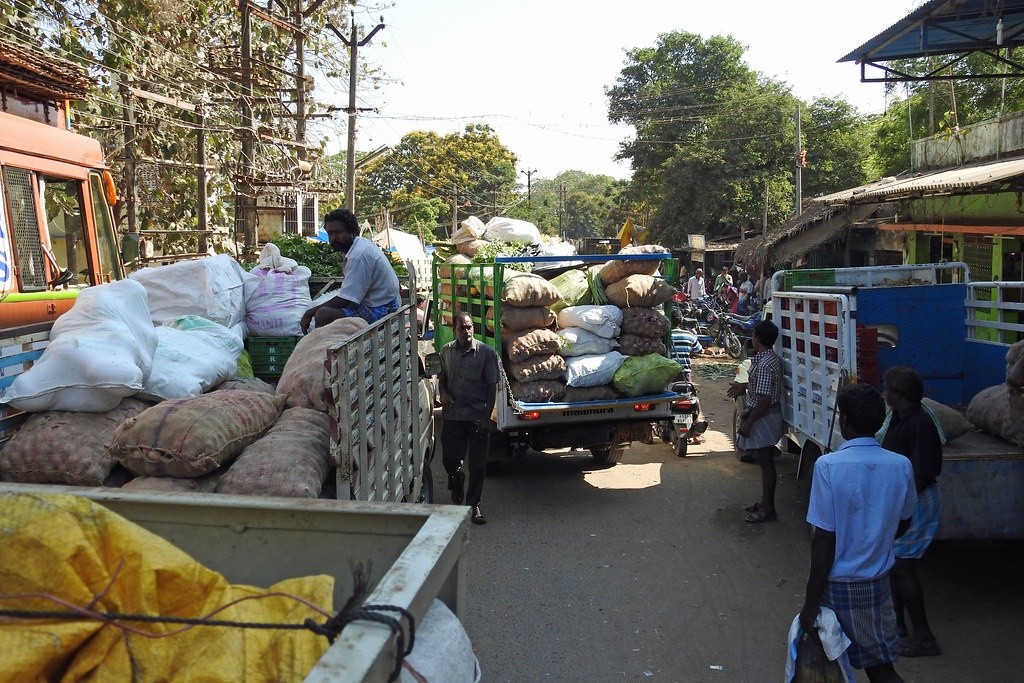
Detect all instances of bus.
[0,111,127,446]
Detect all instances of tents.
[616,219,650,248]
[371,228,425,261]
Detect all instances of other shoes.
[451,471,465,504]
[472,504,487,525]
[896,626,942,656]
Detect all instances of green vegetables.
[474,237,533,272]
[246,230,409,286]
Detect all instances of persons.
[727,320,784,523]
[436,311,501,524]
[881,365,942,657]
[299,208,403,336]
[798,382,919,682]
[669,306,702,443]
[658,261,776,333]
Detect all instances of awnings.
[837,1,1024,63]
[811,156,1024,204]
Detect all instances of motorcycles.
[671,294,763,359]
[656,381,709,458]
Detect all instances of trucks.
[430,241,676,475]
[0,261,433,505]
[760,262,1024,539]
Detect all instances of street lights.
[452,184,472,235]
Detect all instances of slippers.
[744,503,759,513]
[745,513,777,523]
